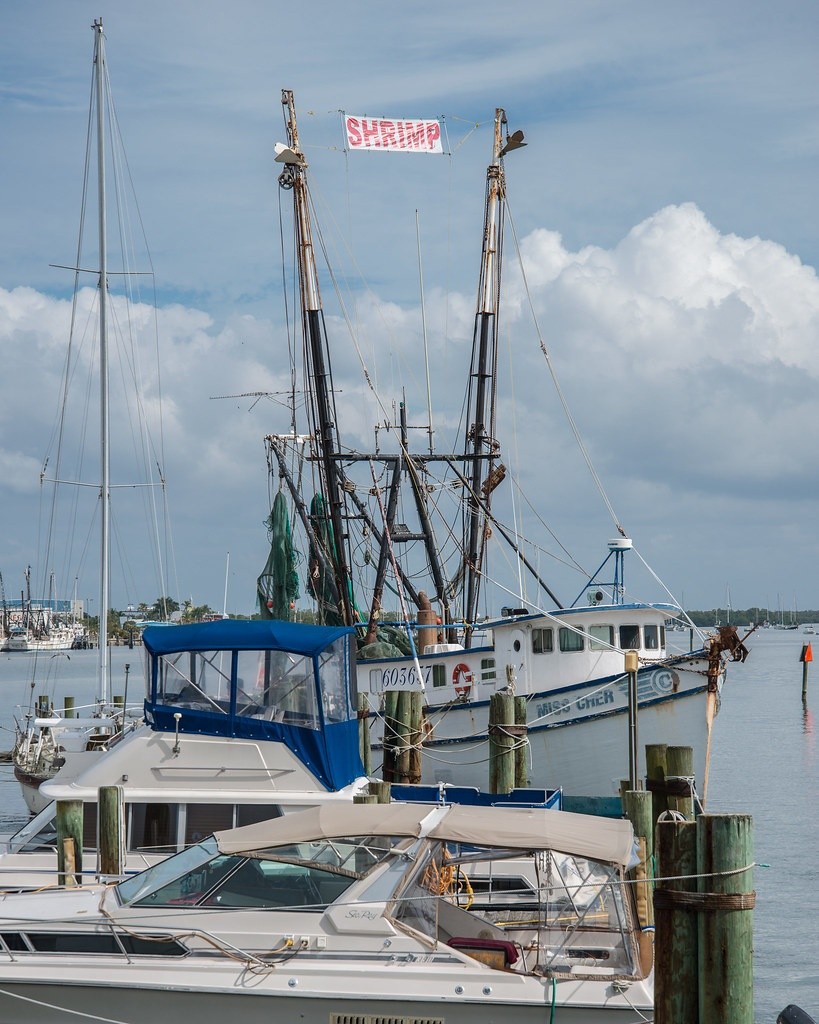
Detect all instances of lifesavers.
[452,663,472,698]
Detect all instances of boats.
[1,798,655,1024]
[0,16,819,1023]
